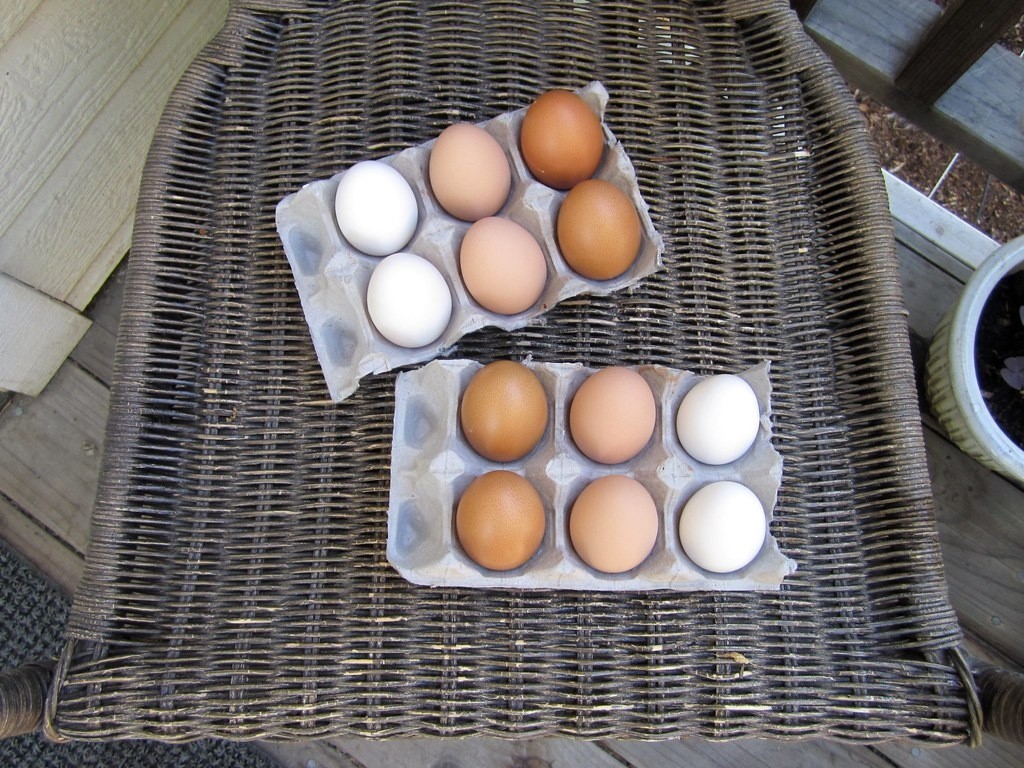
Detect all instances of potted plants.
[923,233,1024,488]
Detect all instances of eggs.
[455,358,767,573]
[333,87,641,347]
